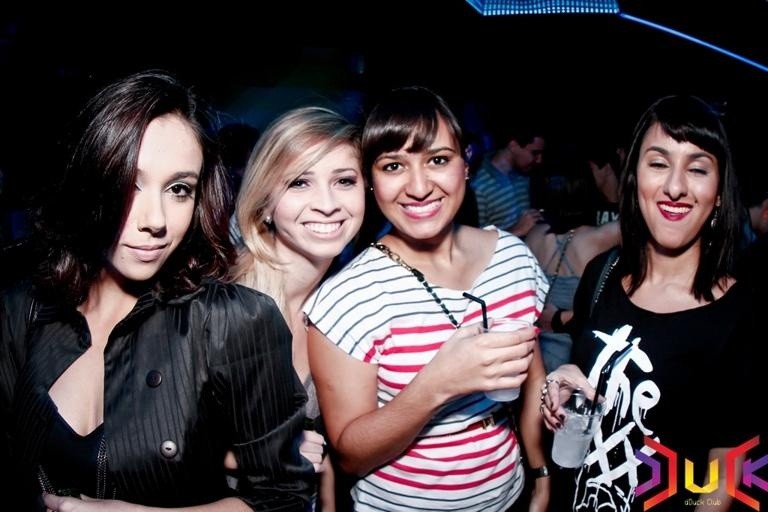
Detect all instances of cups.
[548,384,608,473]
[475,316,532,403]
